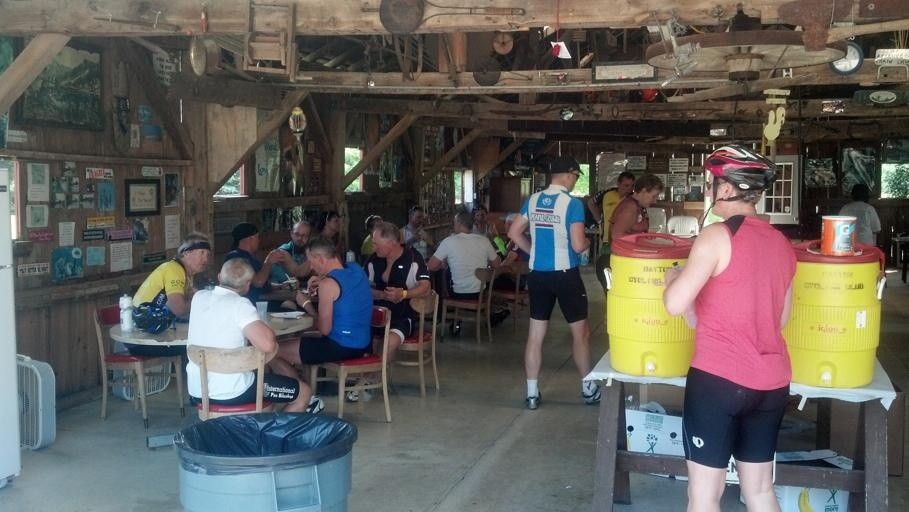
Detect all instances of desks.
[582,348,906,511]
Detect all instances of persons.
[219,210,431,416]
[399,206,531,329]
[587,172,635,253]
[663,142,798,512]
[608,174,664,252]
[837,184,882,246]
[505,156,603,411]
[185,258,312,416]
[131,232,211,405]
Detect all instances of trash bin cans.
[172,411,358,512]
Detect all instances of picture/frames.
[125,179,162,216]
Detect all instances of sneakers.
[582,386,601,403]
[450,319,463,336]
[485,310,511,328]
[346,378,373,401]
[526,392,541,409]
[306,394,325,414]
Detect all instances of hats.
[551,156,584,175]
[233,224,257,239]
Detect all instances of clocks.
[829,40,865,74]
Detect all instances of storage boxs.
[817,379,905,476]
[625,383,689,481]
[740,449,864,511]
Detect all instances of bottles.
[119,293,134,333]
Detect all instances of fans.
[113,340,172,400]
[17,353,56,450]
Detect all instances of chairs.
[373,290,439,396]
[309,305,392,424]
[93,304,185,429]
[188,343,279,422]
[441,267,504,342]
[493,261,529,332]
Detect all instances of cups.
[256,301,268,321]
[289,278,298,291]
[345,252,355,263]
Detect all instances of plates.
[270,310,305,319]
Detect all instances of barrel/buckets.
[604,233,696,378]
[781,240,885,389]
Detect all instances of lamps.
[645,29,848,81]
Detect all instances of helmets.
[704,144,780,192]
[133,303,175,334]
